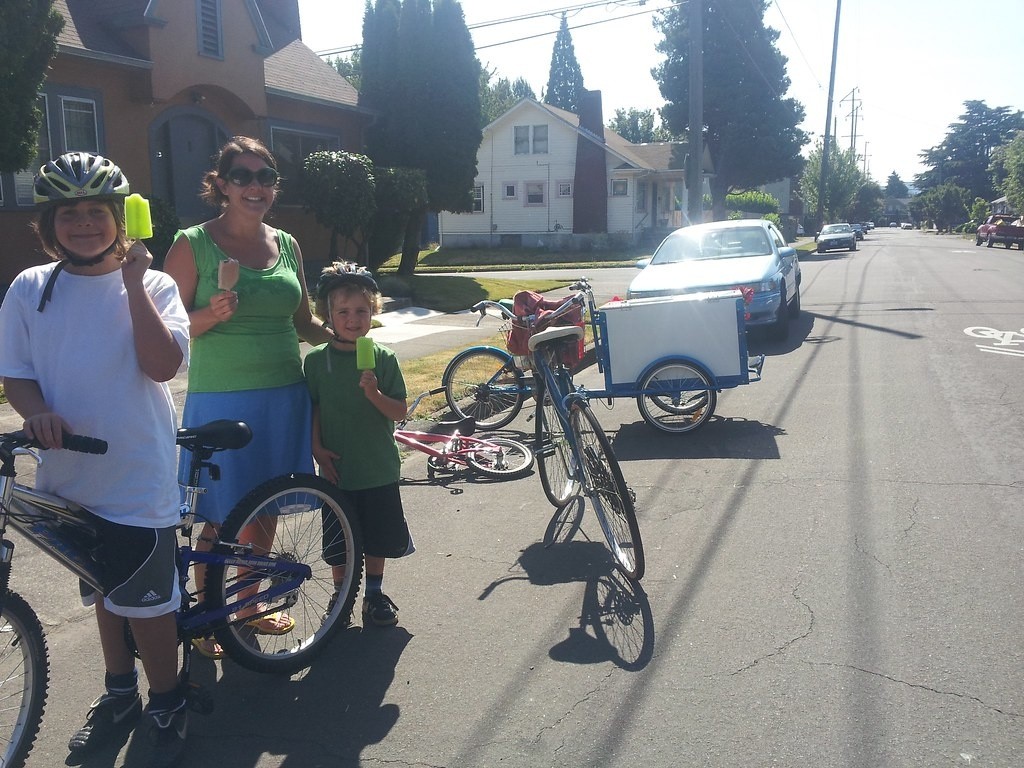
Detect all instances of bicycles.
[391,386,534,481]
[0,419,365,768]
[471,293,646,581]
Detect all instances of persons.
[305,256,416,633]
[162,134,334,660]
[0,152,191,768]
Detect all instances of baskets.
[498,317,587,372]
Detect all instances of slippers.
[192,636,227,659]
[245,614,294,634]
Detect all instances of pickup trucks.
[976,213,1024,251]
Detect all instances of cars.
[816,222,856,253]
[859,221,875,234]
[889,222,897,228]
[850,224,863,240]
[903,223,912,230]
[626,219,801,343]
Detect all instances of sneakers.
[362,595,399,625]
[144,696,188,768]
[69,691,142,752]
[320,593,356,629]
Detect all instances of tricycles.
[441,279,766,439]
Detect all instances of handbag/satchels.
[506,291,582,356]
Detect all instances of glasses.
[226,165,279,188]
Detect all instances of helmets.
[32,152,129,204]
[317,262,378,298]
